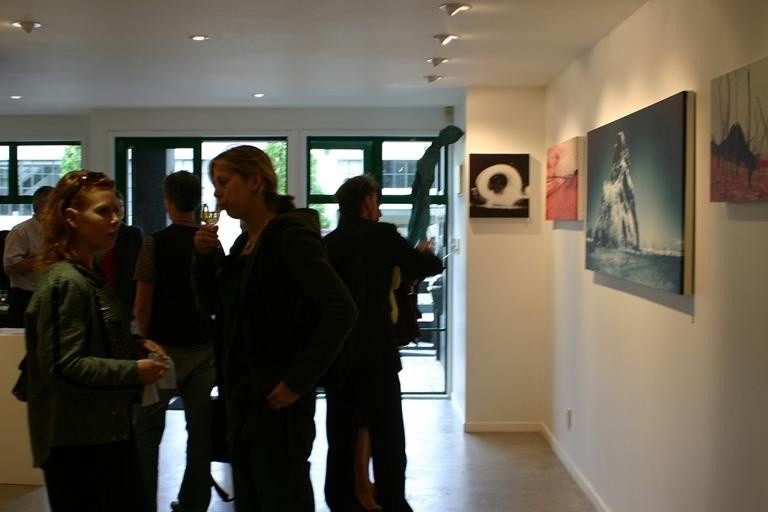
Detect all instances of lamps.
[426,4,471,83]
[11,21,43,34]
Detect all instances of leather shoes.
[171,500,183,512]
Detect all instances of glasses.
[63,172,104,208]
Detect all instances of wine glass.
[200,210,221,227]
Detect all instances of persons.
[611,132,640,251]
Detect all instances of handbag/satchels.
[12,355,29,402]
